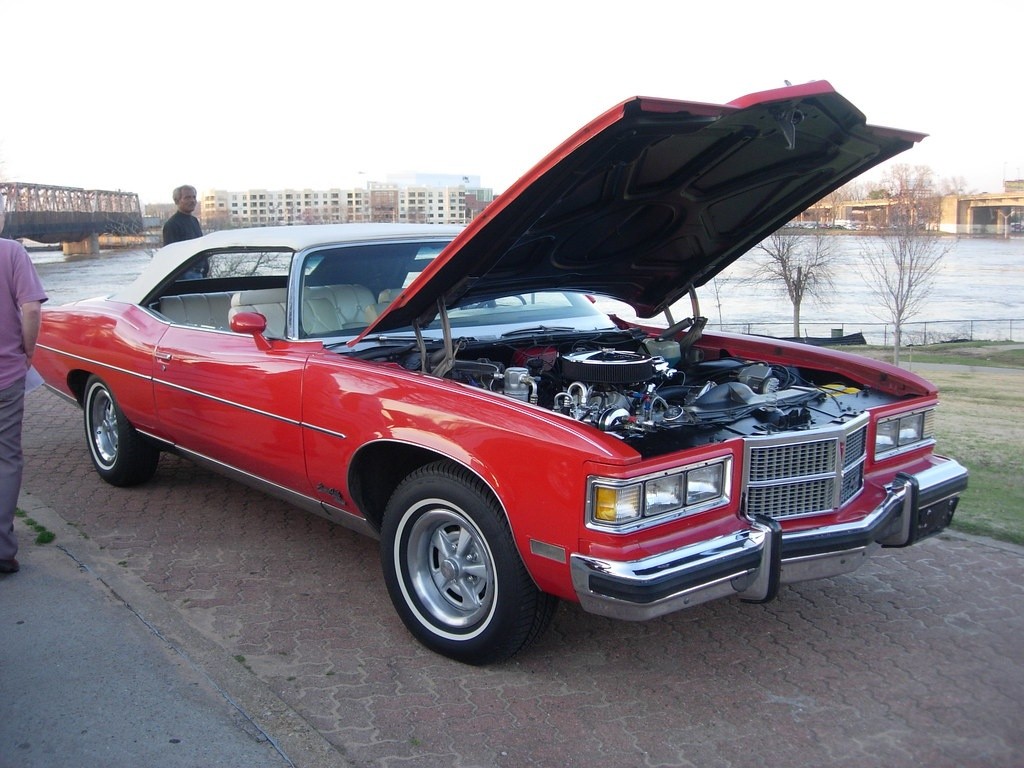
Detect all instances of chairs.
[229,287,343,336]
[364,288,406,326]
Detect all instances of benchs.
[157,283,377,339]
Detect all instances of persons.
[163,186,209,280]
[0,197,49,575]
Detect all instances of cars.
[26,79,970,668]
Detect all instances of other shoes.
[0,558,20,572]
